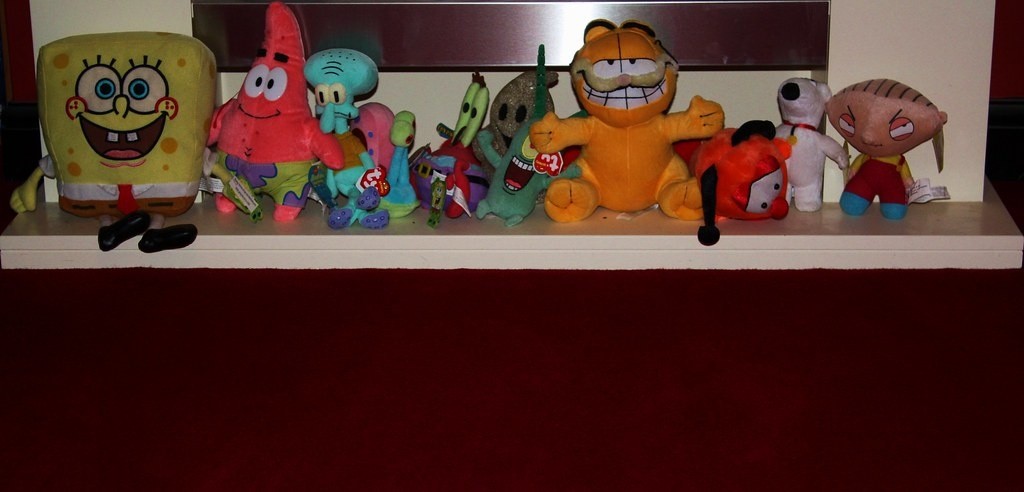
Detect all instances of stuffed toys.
[8,0,947,254]
